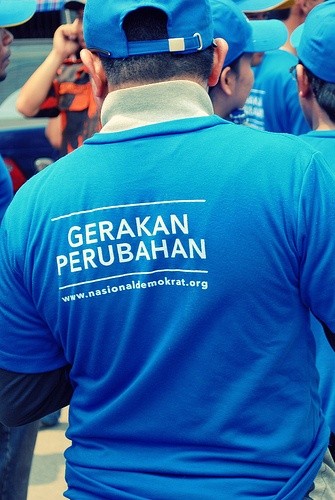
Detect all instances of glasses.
[290,64,297,76]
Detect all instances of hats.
[291,0,335,83]
[82,0,214,58]
[64,0,86,10]
[0,0,37,29]
[208,0,288,70]
[234,0,291,13]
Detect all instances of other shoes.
[41,410,61,428]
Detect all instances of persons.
[0,0,335,500]
